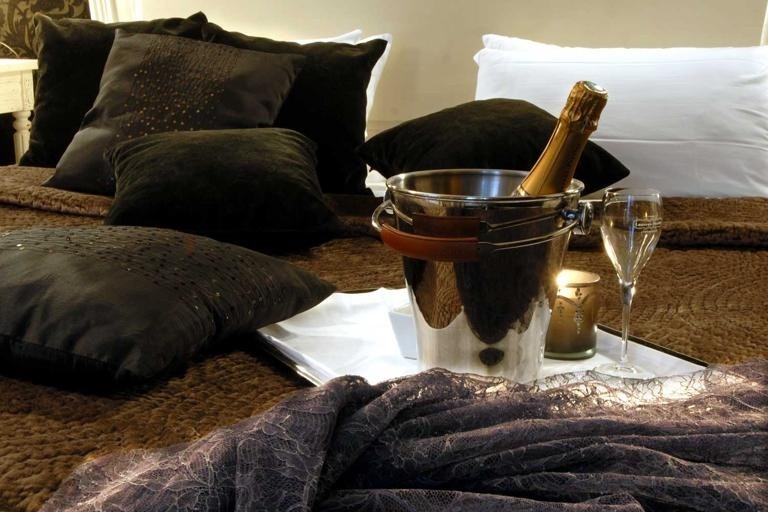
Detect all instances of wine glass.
[592,185,664,381]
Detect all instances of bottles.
[508,80,608,196]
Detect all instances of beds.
[0,0,767,512]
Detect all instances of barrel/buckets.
[370,168,584,382]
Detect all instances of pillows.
[366,93,633,196]
[37,27,315,198]
[302,27,399,138]
[471,30,768,195]
[15,10,210,169]
[173,12,391,195]
[104,126,361,253]
[3,221,338,397]
[361,162,390,197]
